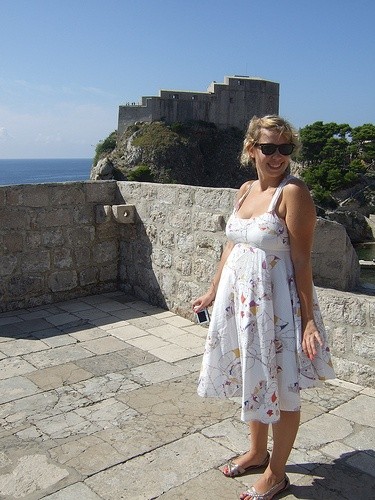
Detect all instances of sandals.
[240,474,290,500]
[223,451,270,477]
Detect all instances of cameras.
[194,305,210,325]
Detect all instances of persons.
[192,115,335,500]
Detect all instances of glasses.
[252,141,296,155]
